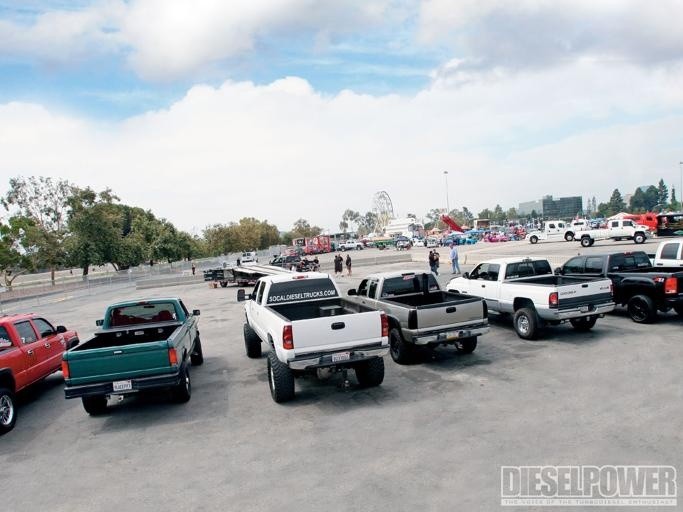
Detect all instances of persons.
[286,256,320,272]
[449,243,462,275]
[191,261,195,274]
[236,257,240,265]
[337,254,342,264]
[429,251,440,277]
[334,255,344,278]
[345,253,352,276]
[432,249,440,268]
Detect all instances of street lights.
[443,171,450,215]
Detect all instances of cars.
[242,252,253,262]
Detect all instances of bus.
[291,234,330,256]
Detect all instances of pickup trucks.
[524,220,575,243]
[237,270,390,405]
[270,254,320,272]
[552,249,683,323]
[444,256,616,342]
[338,239,365,252]
[62,296,205,416]
[0,308,80,436]
[646,238,683,269]
[573,218,650,247]
[340,268,491,365]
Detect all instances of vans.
[568,220,603,230]
[604,212,658,240]
[426,238,436,248]
